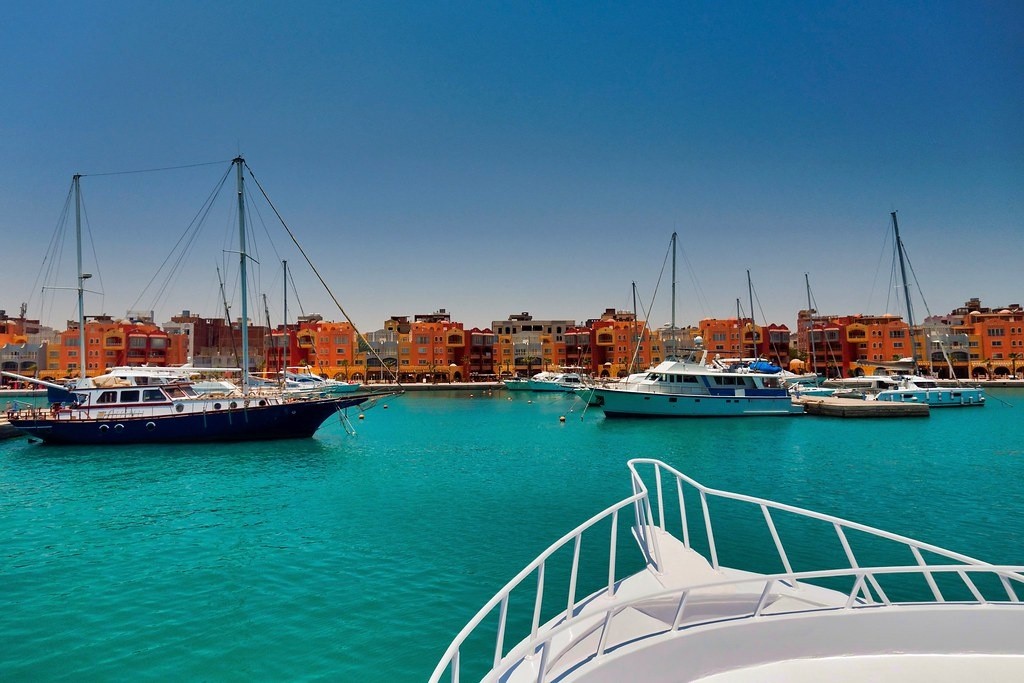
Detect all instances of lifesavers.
[51,405,62,413]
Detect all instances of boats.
[504,371,598,407]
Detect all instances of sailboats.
[716,271,911,397]
[874,211,986,408]
[586,232,808,416]
[8,156,407,446]
